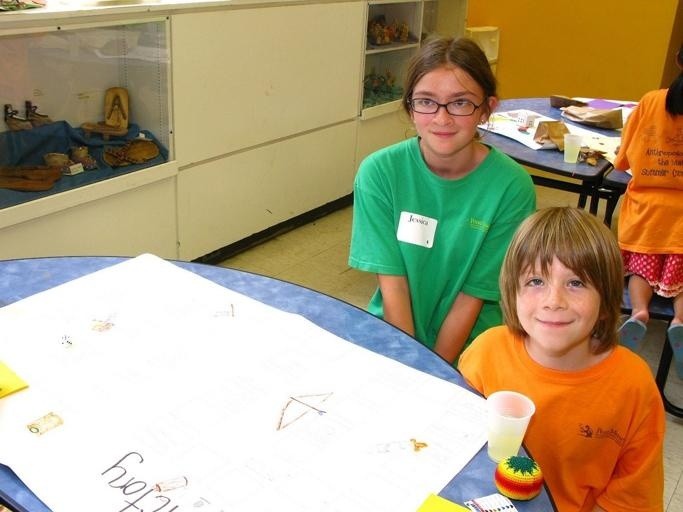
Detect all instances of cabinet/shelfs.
[2,0,464,267]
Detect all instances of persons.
[456,204,667,511]
[345,36,537,369]
[610,44,682,382]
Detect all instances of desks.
[0,252,556,512]
[478,94,643,224]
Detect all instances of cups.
[561,133,584,164]
[483,391,534,463]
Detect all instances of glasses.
[407,97,484,116]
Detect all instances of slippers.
[0,87,159,191]
[668,324,683,380]
[617,319,647,352]
